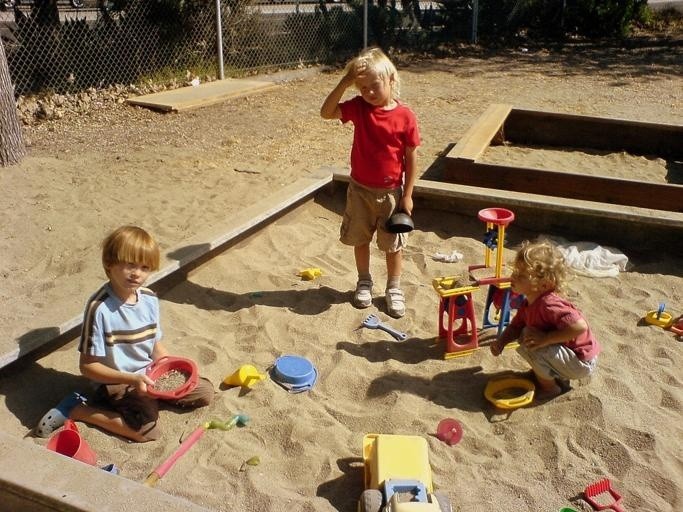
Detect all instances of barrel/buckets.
[46,416,97,466]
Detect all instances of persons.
[33,224,218,445]
[318,46,421,317]
[489,234,600,401]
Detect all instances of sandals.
[34,389,87,438]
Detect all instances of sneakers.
[353,279,373,309]
[385,288,405,318]
